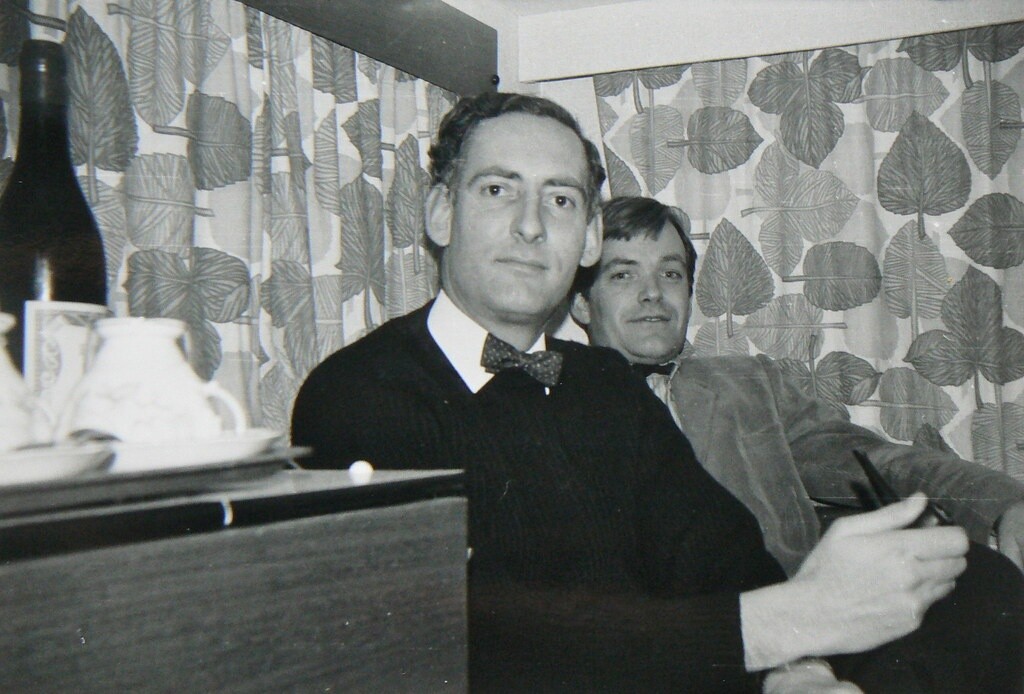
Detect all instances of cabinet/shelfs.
[0,465,469,693]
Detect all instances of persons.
[569,196,1024,694]
[290,93,968,694]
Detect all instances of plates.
[119,422,280,467]
[0,447,111,487]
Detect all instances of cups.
[22,297,110,439]
[86,319,247,446]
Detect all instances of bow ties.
[632,362,675,380]
[480,333,564,385]
